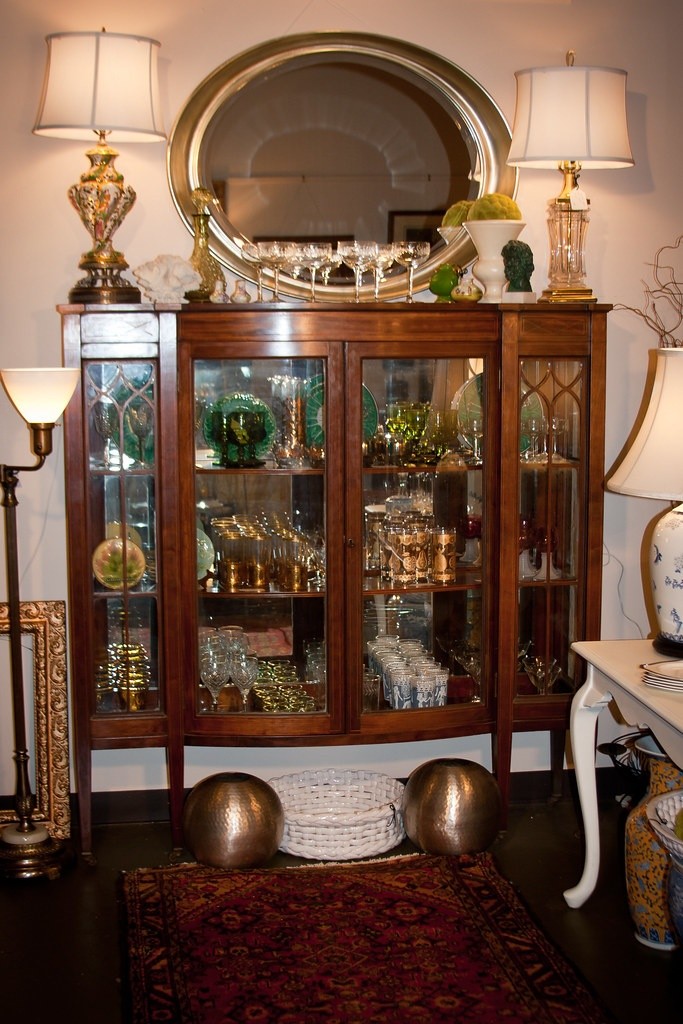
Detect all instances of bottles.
[209,279,229,304]
[451,278,483,303]
[428,263,458,303]
[230,280,251,304]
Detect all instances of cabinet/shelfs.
[57,302,612,859]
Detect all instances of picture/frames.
[0,601,72,846]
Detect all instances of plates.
[92,538,145,589]
[204,393,275,459]
[641,659,683,692]
[452,369,542,459]
[197,530,214,578]
[109,384,156,464]
[306,374,378,453]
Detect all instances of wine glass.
[456,652,482,703]
[198,625,258,657]
[523,656,561,695]
[374,404,565,462]
[241,240,430,302]
[517,639,532,672]
[197,657,258,712]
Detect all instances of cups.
[258,659,298,683]
[302,635,434,683]
[255,683,317,714]
[98,671,150,711]
[314,657,449,713]
[211,510,297,552]
[364,510,431,567]
[107,643,150,670]
[209,527,457,593]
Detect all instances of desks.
[564,637,683,913]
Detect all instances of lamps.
[607,345,683,658]
[507,49,634,297]
[31,29,161,307]
[0,365,81,878]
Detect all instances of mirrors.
[165,29,518,300]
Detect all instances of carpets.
[117,851,613,1024]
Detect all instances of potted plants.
[462,193,523,300]
[438,200,468,244]
